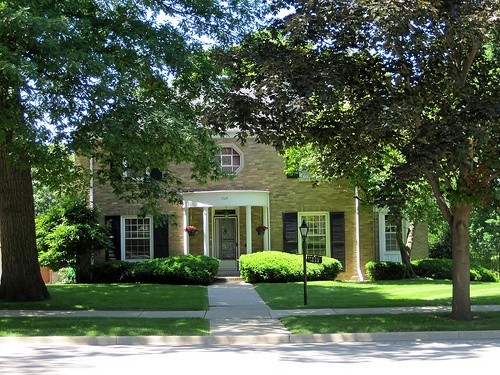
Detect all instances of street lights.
[300,218,309,307]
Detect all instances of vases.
[258,230,265,235]
[189,232,195,236]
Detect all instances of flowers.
[186,225,197,232]
[256,226,268,231]
